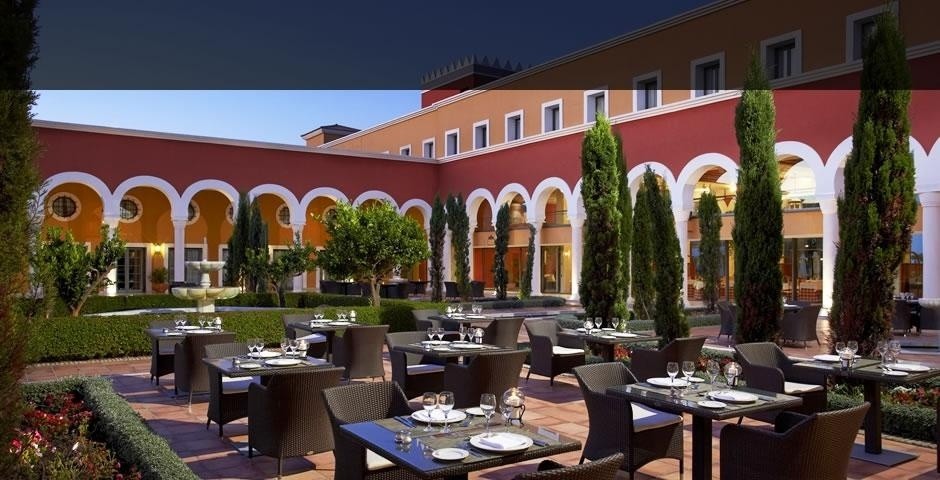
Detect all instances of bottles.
[215,317,222,331]
[231,357,240,373]
[396,443,410,454]
[394,430,412,444]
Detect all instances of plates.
[697,401,727,409]
[241,349,301,369]
[311,318,351,327]
[469,433,533,453]
[421,340,483,351]
[432,448,469,461]
[646,377,691,387]
[882,362,931,376]
[168,324,217,335]
[466,407,495,416]
[411,409,467,424]
[445,314,487,320]
[576,327,637,339]
[681,376,705,383]
[708,390,758,404]
[812,353,861,362]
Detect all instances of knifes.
[395,416,416,428]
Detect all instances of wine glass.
[667,362,679,393]
[706,361,720,391]
[500,397,513,433]
[460,327,474,345]
[247,339,264,362]
[723,365,737,392]
[336,311,347,322]
[314,311,324,325]
[174,315,187,332]
[446,304,484,319]
[682,361,695,383]
[438,392,455,433]
[584,317,627,335]
[423,392,437,432]
[480,394,497,437]
[879,342,901,368]
[280,338,298,359]
[427,328,445,350]
[836,340,859,369]
[199,316,213,331]
